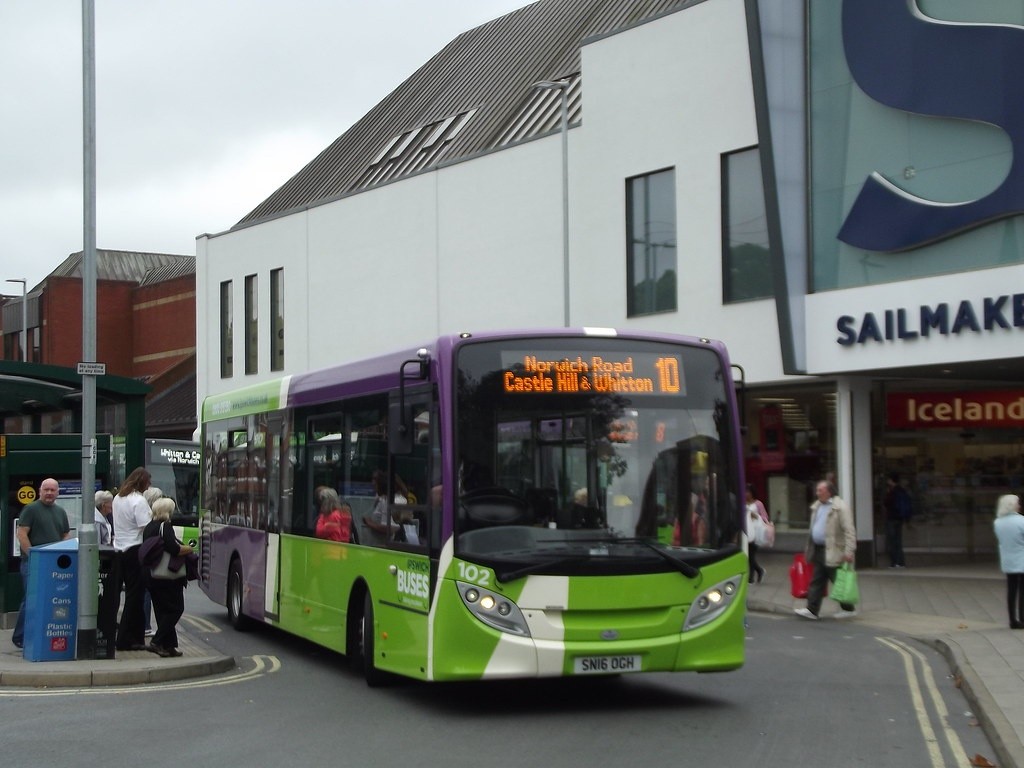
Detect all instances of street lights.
[6,277,27,362]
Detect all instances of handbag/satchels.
[790,553,828,599]
[151,521,186,580]
[755,513,775,548]
[830,560,859,605]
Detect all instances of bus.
[110,436,202,556]
[198,328,753,686]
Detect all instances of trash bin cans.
[21,539,122,662]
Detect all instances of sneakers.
[794,607,819,620]
[834,608,859,619]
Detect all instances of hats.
[889,470,901,484]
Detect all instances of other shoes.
[159,646,183,657]
[1009,620,1024,629]
[13,639,24,648]
[150,640,157,647]
[144,628,155,637]
[114,642,148,650]
[757,567,766,586]
[889,563,905,569]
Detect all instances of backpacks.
[893,491,913,524]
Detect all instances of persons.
[794,480,857,618]
[95,491,120,630]
[111,466,162,651]
[883,474,910,570]
[313,485,351,628]
[993,494,1024,629]
[11,478,70,648]
[363,469,408,543]
[672,492,705,546]
[745,482,768,584]
[143,498,192,658]
